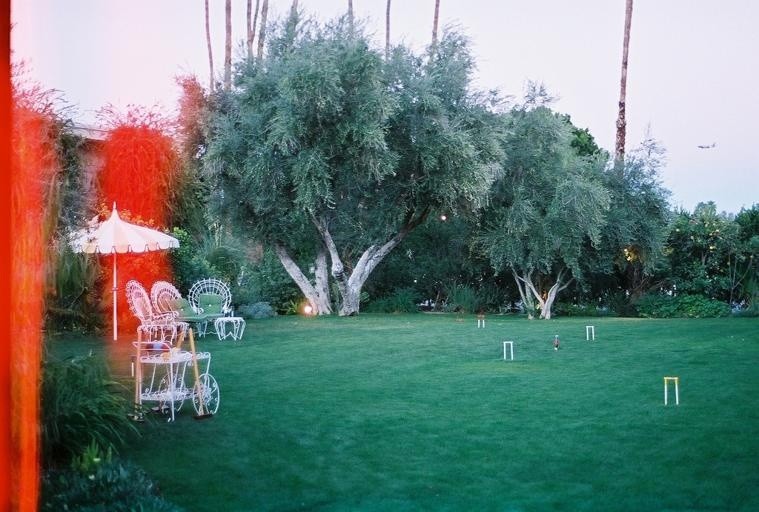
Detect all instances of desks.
[137,351,220,421]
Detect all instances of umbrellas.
[69,200,178,342]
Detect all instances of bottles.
[553,334,560,352]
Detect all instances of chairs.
[124,278,233,347]
